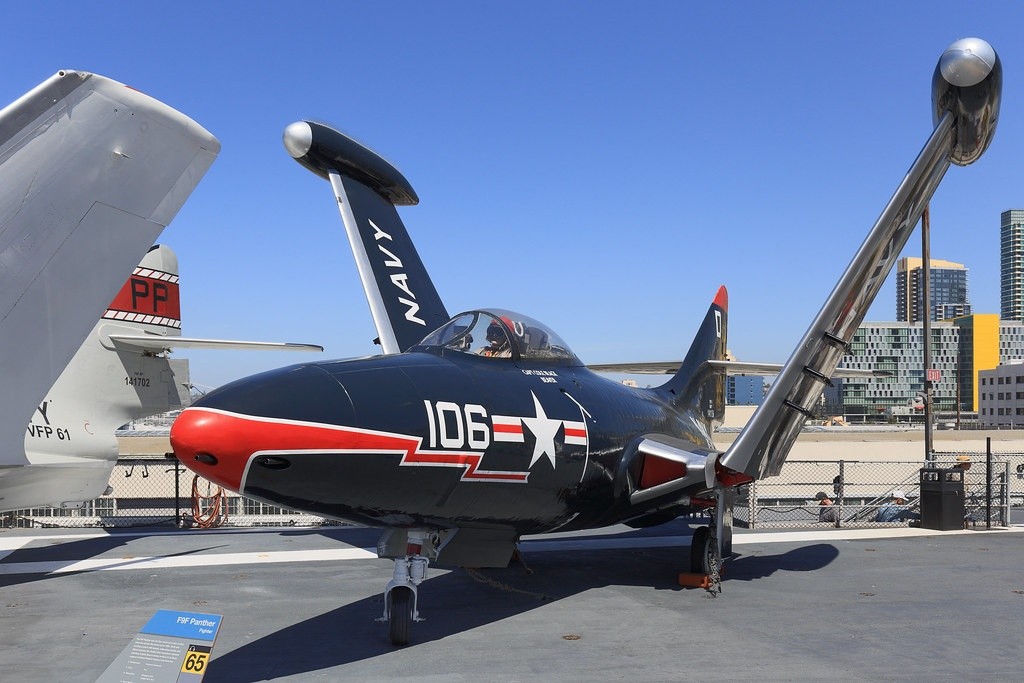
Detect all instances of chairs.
[522,327,548,350]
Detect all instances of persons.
[946,456,974,521]
[814,492,839,523]
[876,490,910,522]
[473,318,514,358]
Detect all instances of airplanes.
[0,68,324,515]
[169,35,1004,650]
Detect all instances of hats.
[813,493,828,502]
[955,456,970,465]
[891,491,909,502]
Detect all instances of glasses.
[487,325,506,338]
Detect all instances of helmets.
[488,316,515,341]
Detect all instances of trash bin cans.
[919,468,964,531]
